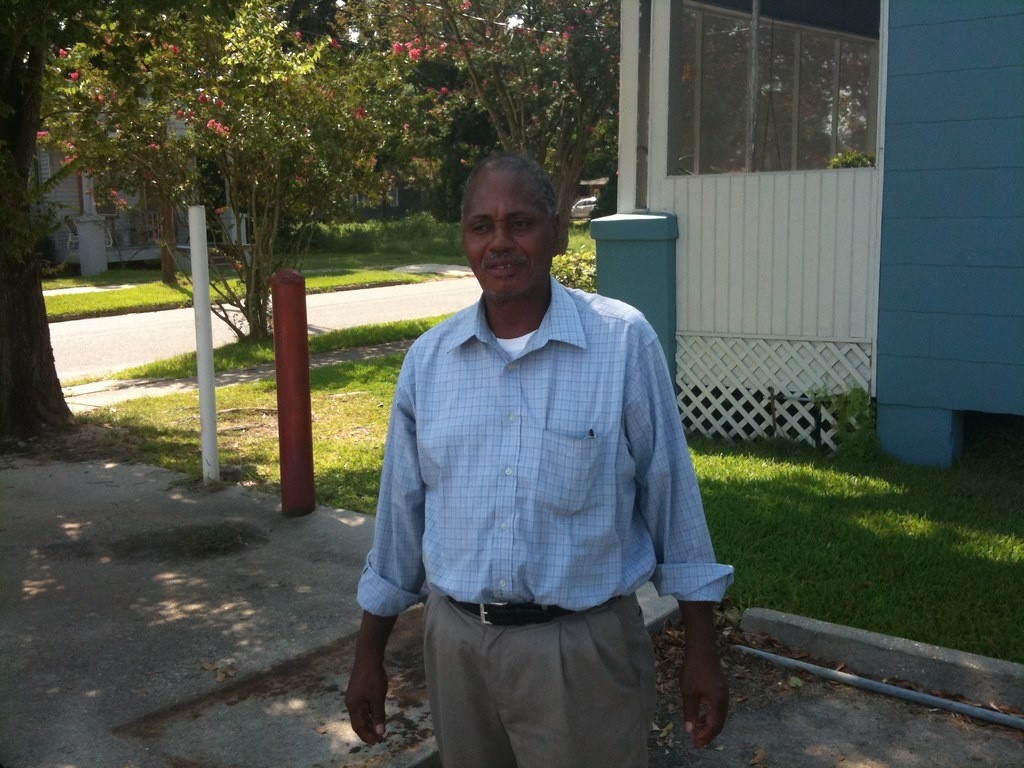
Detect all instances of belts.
[446,596,574,625]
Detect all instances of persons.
[344,152,735,768]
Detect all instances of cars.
[569,197,598,219]
